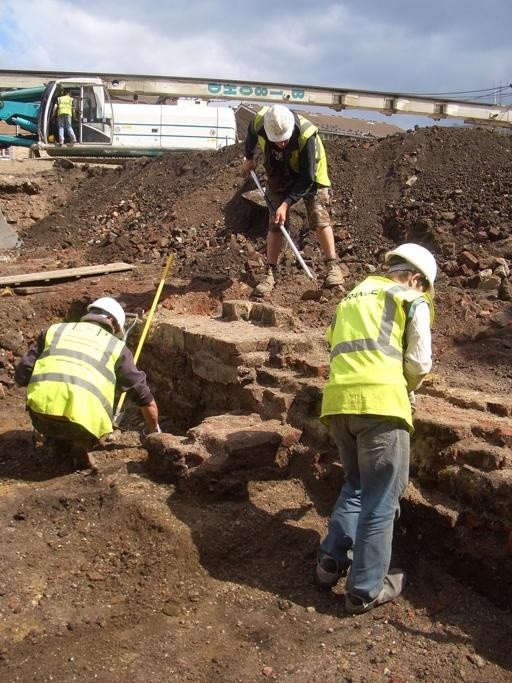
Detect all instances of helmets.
[87,296,126,340]
[384,242,437,299]
[263,103,295,142]
[49,134,56,142]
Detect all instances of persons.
[313,243,438,614]
[13,297,163,475]
[242,104,345,298]
[50,89,78,144]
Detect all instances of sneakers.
[343,568,407,614]
[60,143,68,147]
[253,264,282,296]
[324,257,345,287]
[73,142,80,145]
[316,547,355,587]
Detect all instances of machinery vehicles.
[1,78,238,160]
